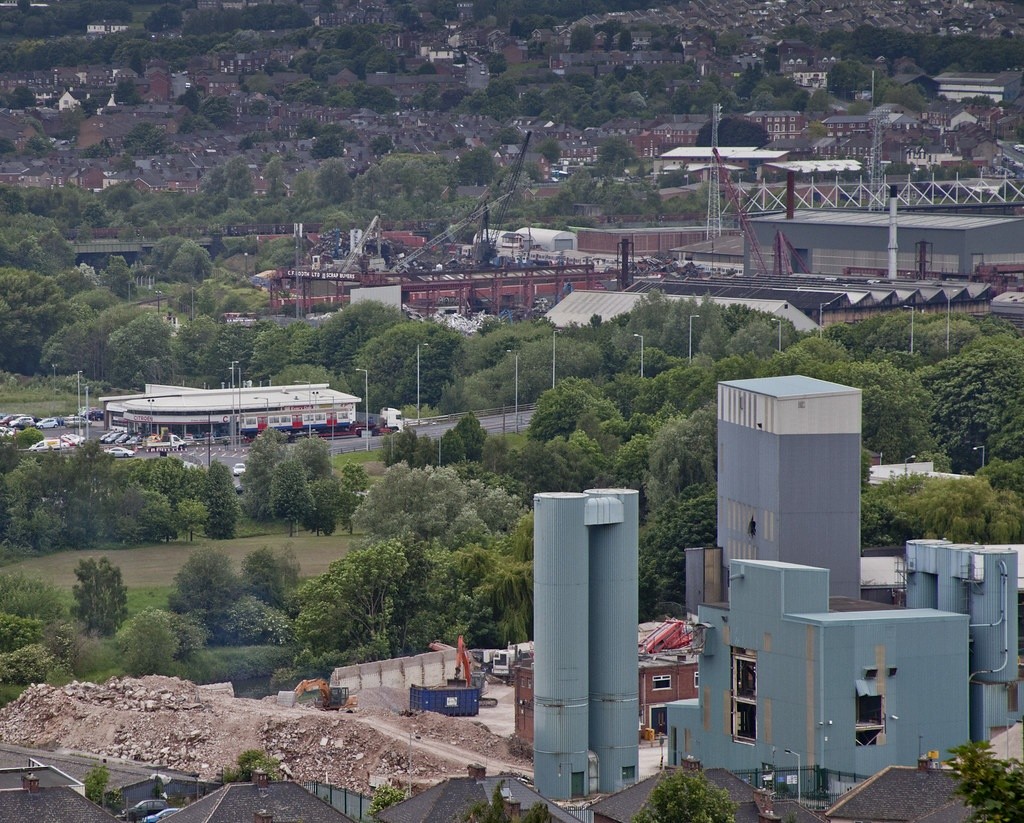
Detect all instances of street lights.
[416,298,916,436]
[52,363,57,400]
[155,291,163,317]
[78,370,83,412]
[230,358,374,452]
[945,289,954,355]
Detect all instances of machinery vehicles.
[430,636,499,708]
[637,617,695,659]
[293,677,359,713]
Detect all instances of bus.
[241,411,353,444]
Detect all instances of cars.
[35,418,58,429]
[78,406,105,422]
[124,799,181,823]
[0,413,38,437]
[51,434,86,448]
[232,462,247,476]
[98,430,161,444]
[64,417,92,427]
[234,484,246,493]
[52,417,63,426]
[109,446,136,458]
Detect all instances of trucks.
[348,407,404,440]
[468,640,534,679]
[142,434,187,453]
[28,439,61,451]
